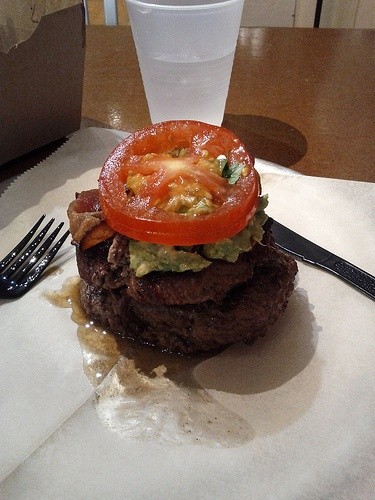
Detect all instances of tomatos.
[99,120,260,246]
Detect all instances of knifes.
[260,214,375,302]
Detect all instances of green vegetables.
[213,152,245,185]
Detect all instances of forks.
[0,212,71,300]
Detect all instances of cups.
[125,0,244,126]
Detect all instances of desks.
[0,24,375,185]
